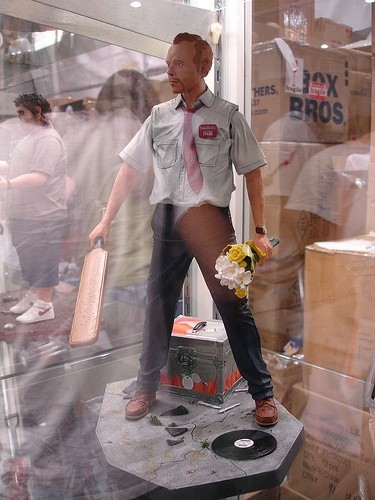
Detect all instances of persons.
[0,94,68,322]
[65,69,162,303]
[261,112,375,358]
[90,32,278,426]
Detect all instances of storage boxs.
[252,0,375,500]
[159,314,245,404]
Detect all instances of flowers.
[214,235,280,299]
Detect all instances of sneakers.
[10,292,39,313]
[16,300,55,323]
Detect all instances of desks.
[94,376,304,500]
